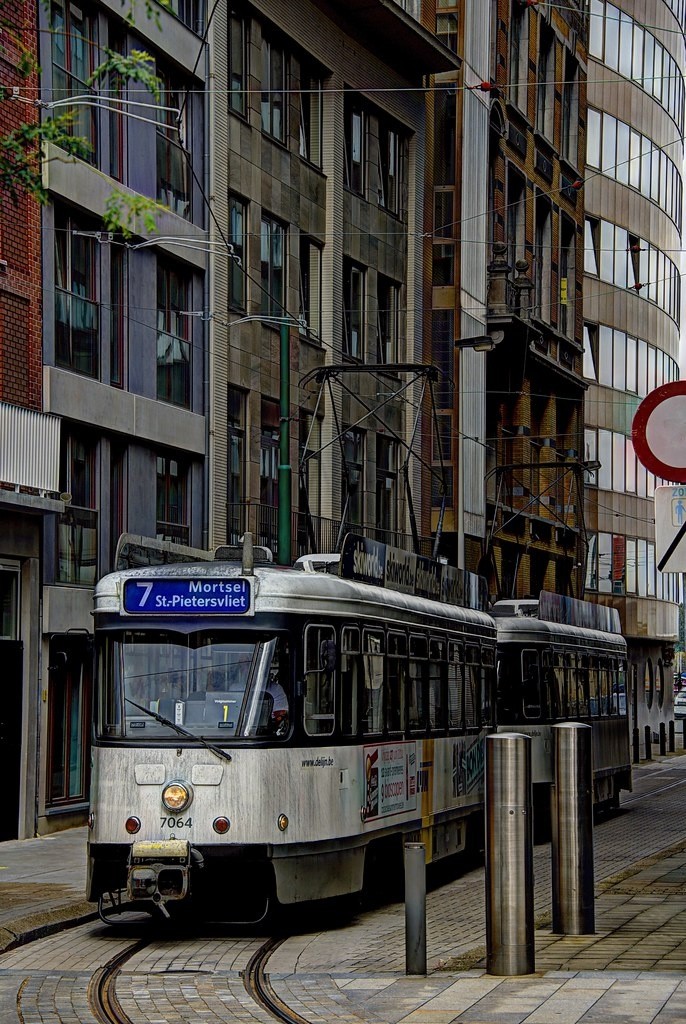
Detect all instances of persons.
[229,652,290,724]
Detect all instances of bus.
[84,528,635,930]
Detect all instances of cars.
[673,692,686,718]
